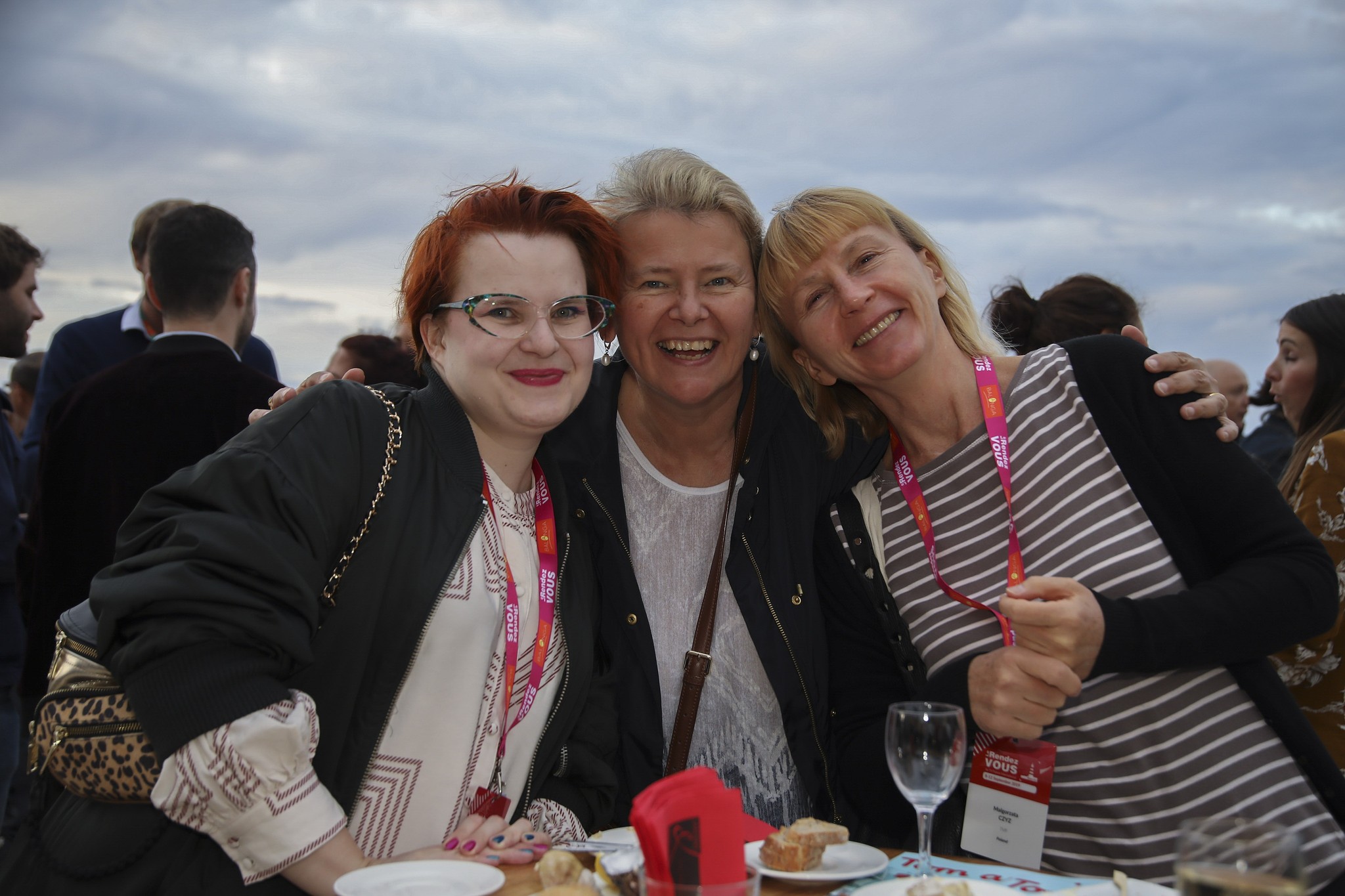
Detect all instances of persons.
[1264,294,1345,778]
[4,351,46,422]
[21,204,288,699]
[991,274,1150,357]
[756,187,1345,896]
[0,220,46,896]
[0,169,621,896]
[1239,378,1298,488]
[326,334,405,386]
[1199,360,1250,437]
[12,199,280,531]
[247,146,1239,852]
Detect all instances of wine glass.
[883,699,969,877]
[1177,812,1305,896]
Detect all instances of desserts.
[528,850,600,896]
[906,877,976,896]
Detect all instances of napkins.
[629,766,779,896]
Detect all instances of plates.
[850,877,1023,896]
[332,860,505,896]
[587,826,641,855]
[743,838,888,883]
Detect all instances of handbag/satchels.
[24,595,166,809]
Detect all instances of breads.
[759,817,849,871]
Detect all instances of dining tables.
[483,847,1065,896]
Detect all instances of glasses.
[431,293,616,341]
